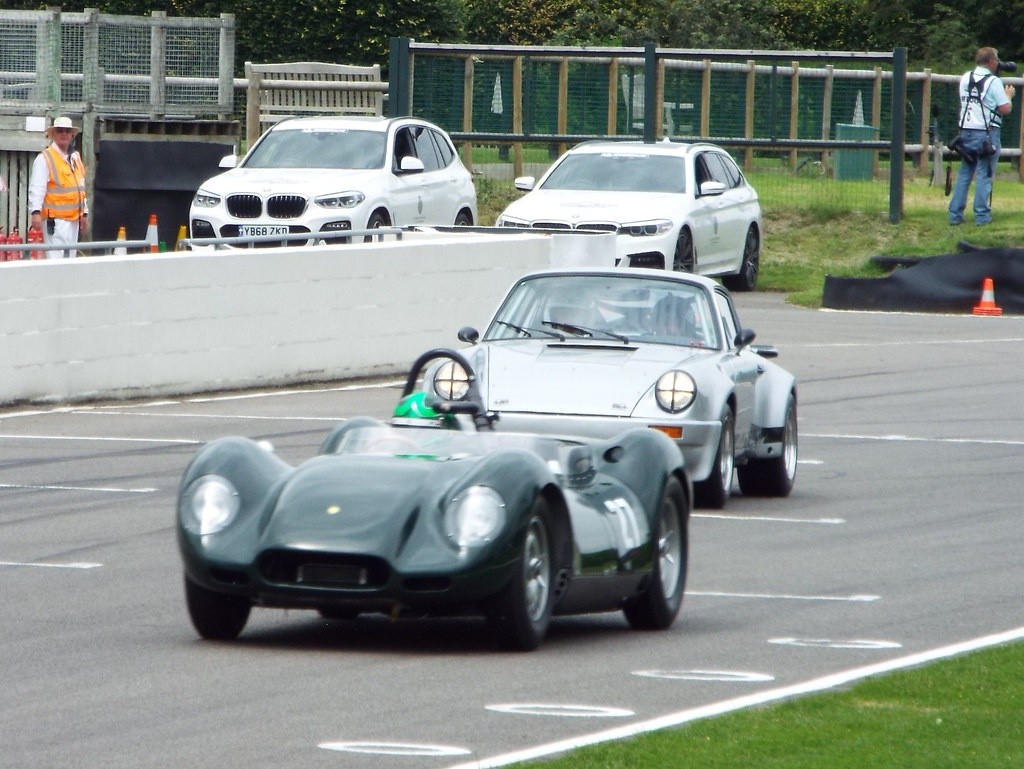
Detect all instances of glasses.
[54,129,72,134]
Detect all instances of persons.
[29,117,89,258]
[549,297,593,333]
[651,305,684,337]
[948,46,1016,227]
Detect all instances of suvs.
[494,140,764,297]
[189,114,478,253]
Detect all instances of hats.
[48,117,78,139]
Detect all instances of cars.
[421,268,803,510]
[173,344,695,653]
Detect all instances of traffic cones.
[139,213,160,254]
[111,227,129,255]
[972,277,1002,316]
[173,226,188,253]
